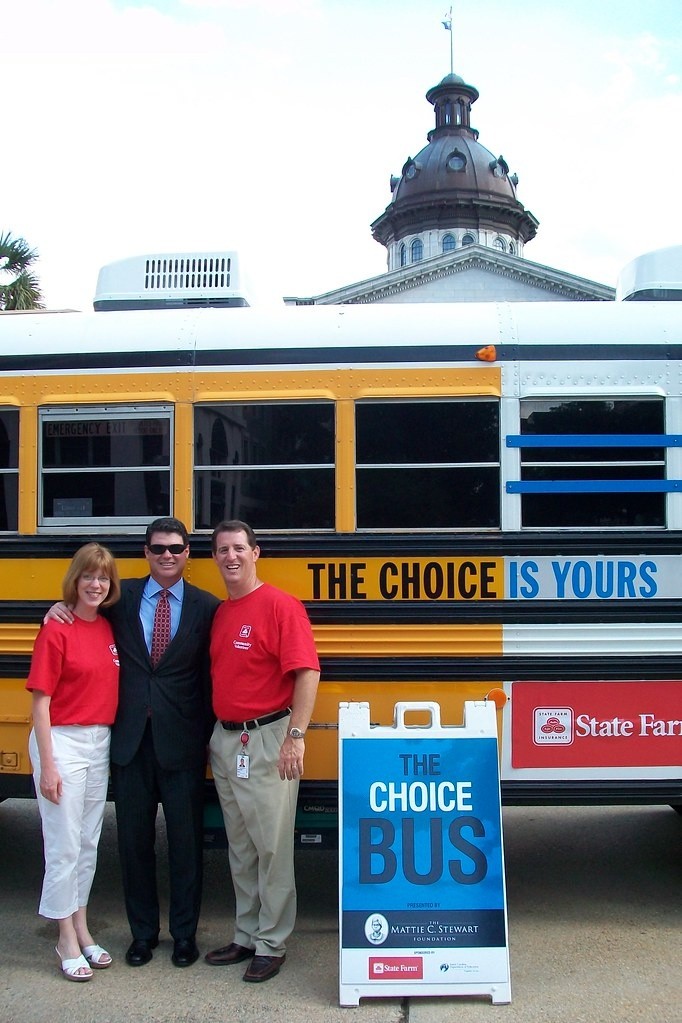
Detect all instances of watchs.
[287,725,306,739]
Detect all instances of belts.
[221,705,294,731]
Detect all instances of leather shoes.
[243,954,286,982]
[205,942,256,966]
[126,935,159,965]
[171,937,199,966]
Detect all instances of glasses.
[81,573,109,582]
[148,544,188,555]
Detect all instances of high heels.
[54,945,94,981]
[79,942,112,969]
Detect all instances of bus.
[0,296,681,813]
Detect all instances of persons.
[203,519,321,985]
[41,516,224,968]
[27,542,121,984]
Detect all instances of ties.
[147,590,172,717]
